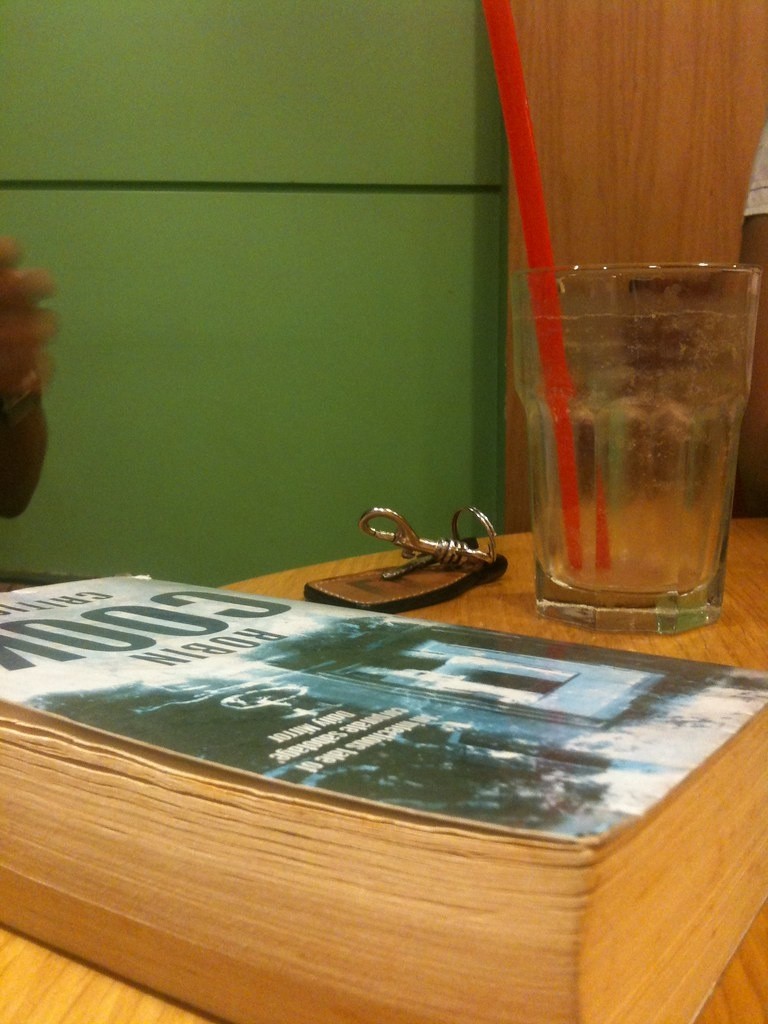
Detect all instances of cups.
[508,263,763,634]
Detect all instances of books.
[0,576,768,1024]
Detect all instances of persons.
[0,234,59,518]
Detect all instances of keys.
[379,533,479,583]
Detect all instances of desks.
[0,512,768,1024]
